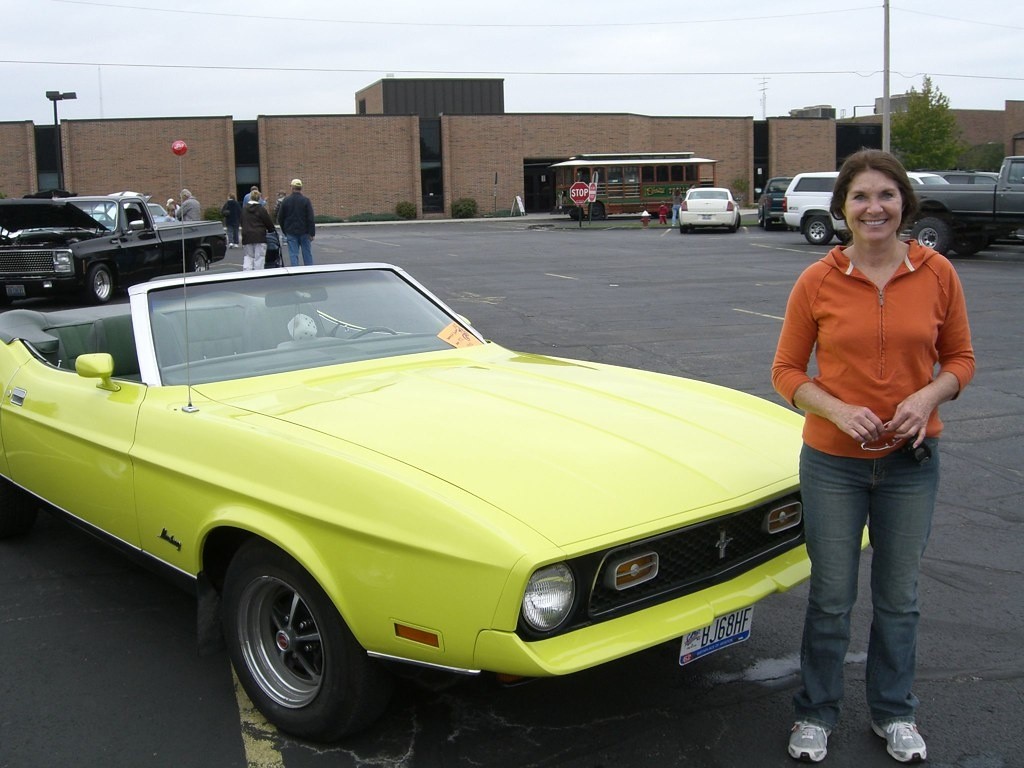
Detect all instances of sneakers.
[234,244,238,248]
[788,719,832,763]
[228,242,233,249]
[870,720,927,763]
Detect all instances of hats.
[166,198,174,206]
[290,178,302,188]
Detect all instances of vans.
[783,171,950,246]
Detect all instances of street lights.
[45,91,77,189]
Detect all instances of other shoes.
[672,224,674,227]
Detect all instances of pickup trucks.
[911,156,1024,255]
[754,176,794,231]
[0,197,229,304]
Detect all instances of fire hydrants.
[640,210,651,229]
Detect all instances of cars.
[83,192,179,229]
[928,169,1000,184]
[678,187,741,233]
[0,262,871,743]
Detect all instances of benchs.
[43,304,245,373]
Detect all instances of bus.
[548,152,718,221]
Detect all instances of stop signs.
[589,183,596,201]
[570,182,590,204]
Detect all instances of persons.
[657,202,668,225]
[772,151,975,764]
[671,183,696,226]
[221,179,315,271]
[166,189,201,221]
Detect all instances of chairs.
[243,301,327,352]
[88,313,186,377]
[114,208,142,227]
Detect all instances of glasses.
[861,421,903,451]
